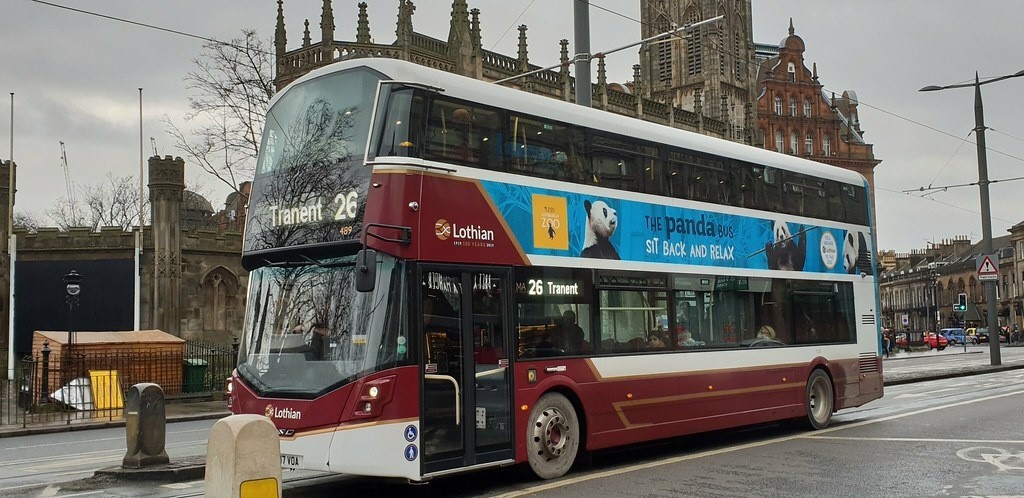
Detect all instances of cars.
[885,327,1010,353]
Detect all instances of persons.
[677,302,699,344]
[883,336,891,359]
[545,311,584,355]
[647,331,668,349]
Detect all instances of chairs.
[456,130,772,211]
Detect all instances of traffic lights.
[958,294,967,312]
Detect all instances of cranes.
[59,141,76,227]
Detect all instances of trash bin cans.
[183,358,208,394]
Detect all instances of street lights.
[63,267,83,424]
[919,68,1024,366]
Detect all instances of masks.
[756,332,763,338]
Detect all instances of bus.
[227,56,886,487]
